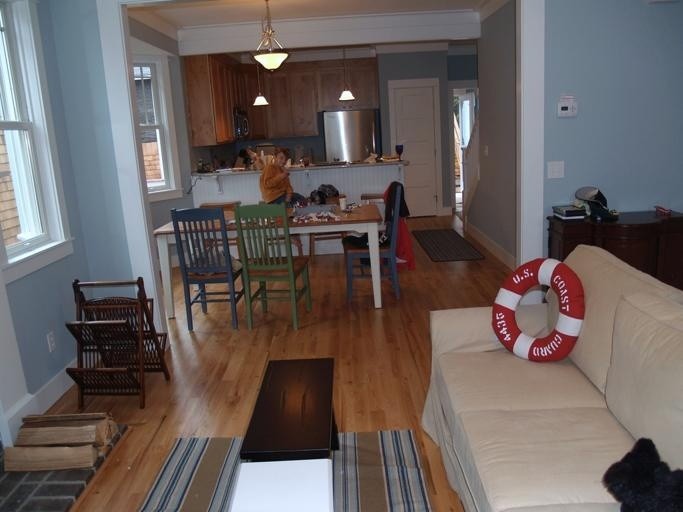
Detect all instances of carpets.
[413,229,485,262]
[136,427,433,511]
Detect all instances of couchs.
[420,243,682,512]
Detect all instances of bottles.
[197,159,203,173]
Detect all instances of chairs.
[152,182,410,331]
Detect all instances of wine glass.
[394,144,406,162]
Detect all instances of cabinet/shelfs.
[318,59,379,112]
[261,62,318,137]
[542,208,683,290]
[182,54,246,147]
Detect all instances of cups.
[339,198,347,211]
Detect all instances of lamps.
[338,45,355,101]
[252,57,270,106]
[254,0,289,71]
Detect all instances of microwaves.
[232,107,250,142]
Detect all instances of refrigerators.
[322,109,380,164]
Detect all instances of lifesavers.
[492,258,584,361]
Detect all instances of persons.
[260,146,304,210]
